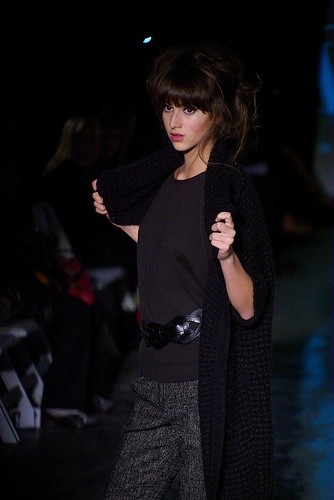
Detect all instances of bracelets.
[217,248,233,260]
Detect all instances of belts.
[143,309,201,351]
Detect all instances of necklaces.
[181,146,207,181]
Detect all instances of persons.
[0,109,334,433]
[88,51,280,500]
